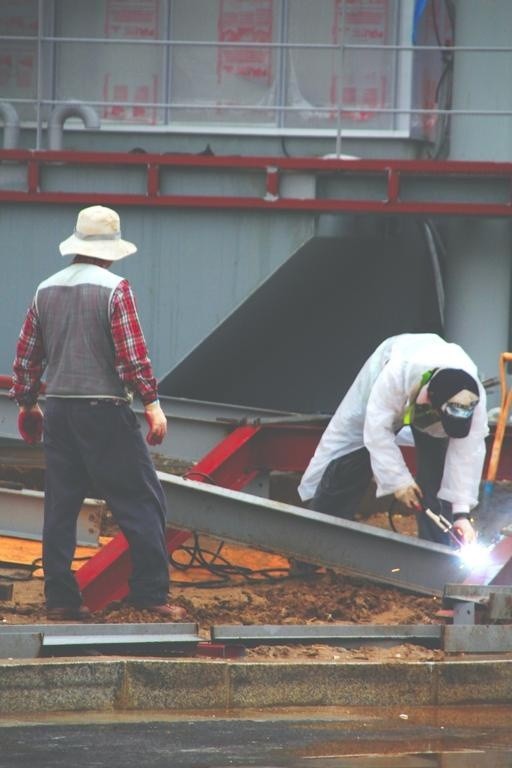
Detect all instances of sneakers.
[124,601,187,620]
[47,605,90,618]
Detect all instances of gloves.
[394,482,423,510]
[454,518,477,545]
[144,399,167,445]
[18,402,44,444]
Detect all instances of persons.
[286,333,489,576]
[7,204,185,622]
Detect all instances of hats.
[59,205,137,261]
[430,369,479,437]
